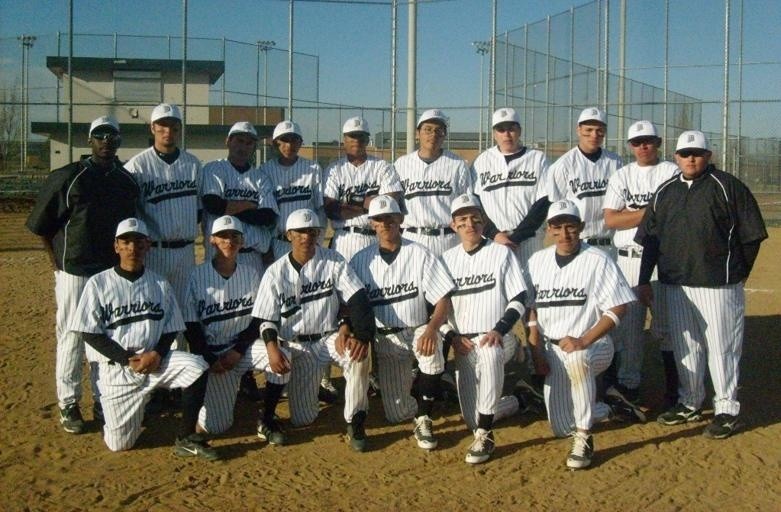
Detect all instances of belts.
[579,238,611,246]
[149,240,195,248]
[238,247,254,253]
[277,234,291,243]
[342,226,376,235]
[212,340,236,352]
[461,332,487,340]
[550,340,559,345]
[503,230,536,238]
[407,227,454,236]
[377,327,404,335]
[297,332,327,342]
[619,250,642,258]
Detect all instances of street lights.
[254,38,277,125]
[470,38,493,155]
[15,32,38,174]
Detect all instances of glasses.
[424,126,444,134]
[631,137,656,146]
[93,131,117,139]
[680,149,704,157]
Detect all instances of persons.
[121,99,682,406]
[632,129,770,440]
[183,214,293,447]
[70,218,228,463]
[429,192,531,465]
[250,207,374,455]
[26,114,143,435]
[520,198,640,470]
[333,192,456,452]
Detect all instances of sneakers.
[321,378,339,397]
[368,372,381,394]
[657,392,703,425]
[465,428,495,463]
[438,373,459,403]
[605,383,647,423]
[175,434,233,461]
[567,435,593,469]
[703,413,740,439]
[345,410,370,452]
[59,402,104,434]
[256,421,289,445]
[513,375,547,416]
[413,417,438,449]
[240,377,261,401]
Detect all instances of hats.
[676,130,708,151]
[286,208,324,232]
[212,215,243,236]
[628,120,657,141]
[417,109,448,128]
[368,195,401,218]
[89,116,120,138]
[577,107,607,126]
[547,200,581,221]
[115,218,149,239]
[228,122,259,141]
[450,193,482,217]
[273,121,303,141]
[151,104,181,124]
[343,117,370,136]
[492,107,520,128]
